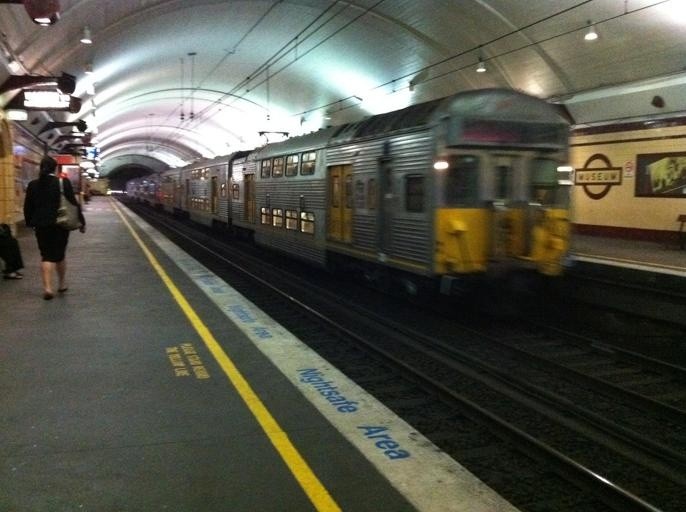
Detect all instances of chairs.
[6,270,23,280]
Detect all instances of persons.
[0,223,25,279]
[23,155,86,300]
[666,160,682,187]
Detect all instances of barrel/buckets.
[58,288,66,292]
[45,295,52,300]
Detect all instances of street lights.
[55,196,82,230]
